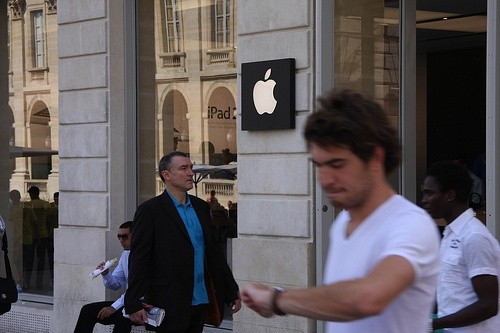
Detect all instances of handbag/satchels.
[0,277,18,316]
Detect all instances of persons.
[240,88,442,333]
[8,186,59,292]
[73,219,144,333]
[432,149,484,205]
[124,151,242,333]
[419,161,500,333]
[207,190,219,208]
[228,200,236,210]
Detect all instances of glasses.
[117,234,131,241]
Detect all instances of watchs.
[270,286,287,318]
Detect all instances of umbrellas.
[191,162,237,198]
[10,146,59,158]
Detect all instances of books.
[122,302,165,326]
